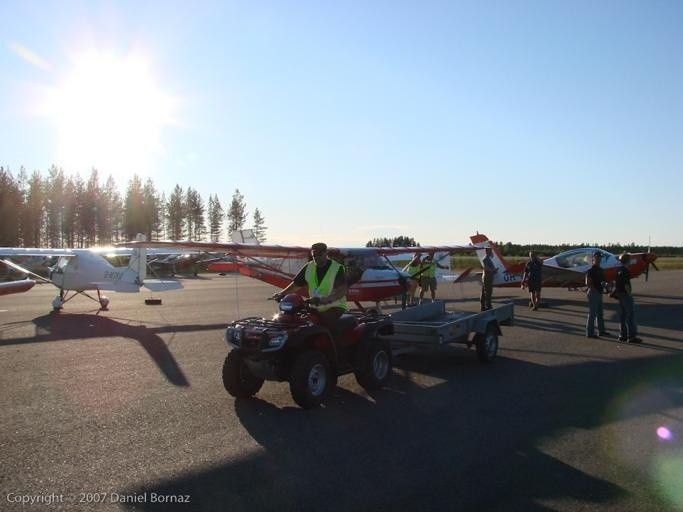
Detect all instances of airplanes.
[0,230,264,312]
[114,227,493,310]
[441,232,660,298]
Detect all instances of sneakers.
[587,331,642,344]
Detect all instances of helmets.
[618,253,631,261]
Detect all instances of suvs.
[222,292,395,406]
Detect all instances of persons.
[520,249,543,311]
[418,251,449,305]
[274,241,350,373]
[521,272,533,308]
[402,252,422,307]
[479,247,499,311]
[610,252,642,344]
[584,251,611,338]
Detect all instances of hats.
[311,243,326,252]
